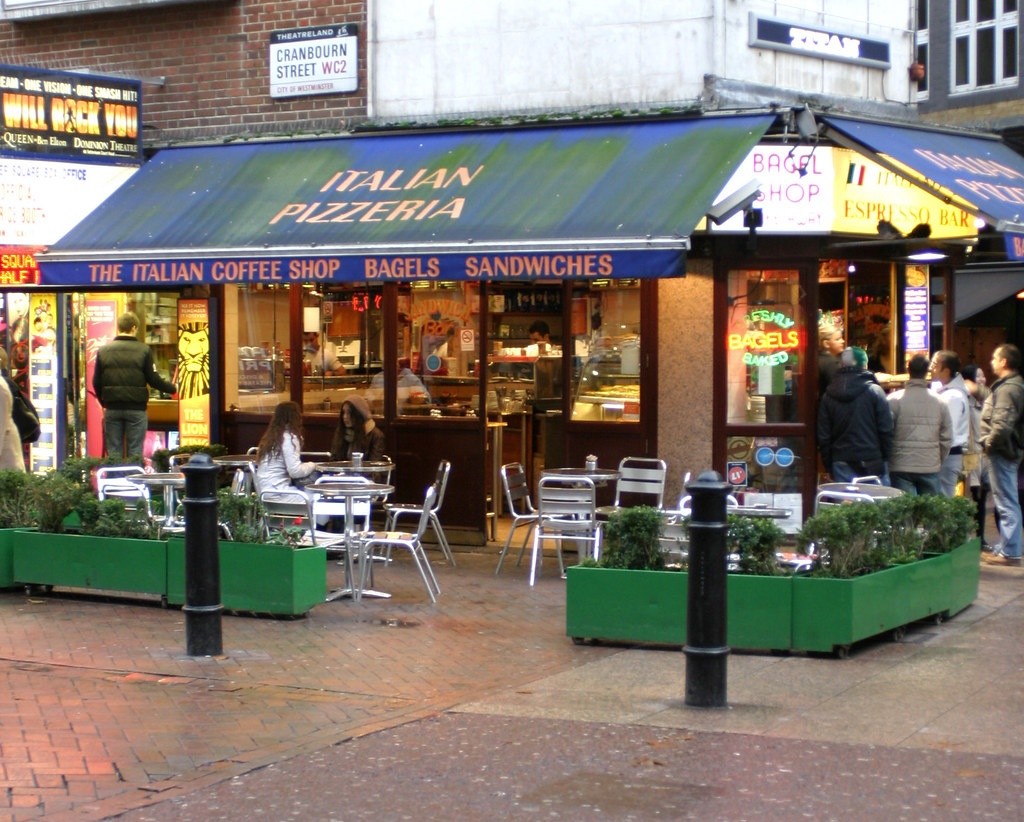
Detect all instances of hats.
[842,346,868,368]
[342,394,372,421]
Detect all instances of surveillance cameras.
[706,178,762,225]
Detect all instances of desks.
[315,461,395,488]
[816,482,906,503]
[212,455,263,496]
[127,474,189,529]
[540,467,622,520]
[304,484,394,601]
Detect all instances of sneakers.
[985,556,1021,565]
[981,552,997,559]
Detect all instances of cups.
[585,461,598,471]
[352,452,361,466]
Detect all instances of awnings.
[823,116,1024,260]
[34,115,776,287]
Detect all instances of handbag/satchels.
[8,379,41,443]
[964,453,979,470]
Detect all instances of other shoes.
[982,544,993,550]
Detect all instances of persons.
[9,293,30,345]
[256,401,345,560]
[303,332,346,376]
[887,353,953,496]
[819,323,909,395]
[966,343,1024,567]
[330,396,385,533]
[366,350,431,403]
[818,346,893,485]
[929,349,969,498]
[92,312,176,458]
[529,320,561,345]
[0,347,26,474]
[33,314,56,354]
[961,365,993,553]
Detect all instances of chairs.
[529,476,599,586]
[496,463,564,579]
[598,457,667,517]
[97,447,457,608]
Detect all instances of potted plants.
[790,493,951,658]
[0,467,332,621]
[567,507,790,655]
[915,494,982,619]
